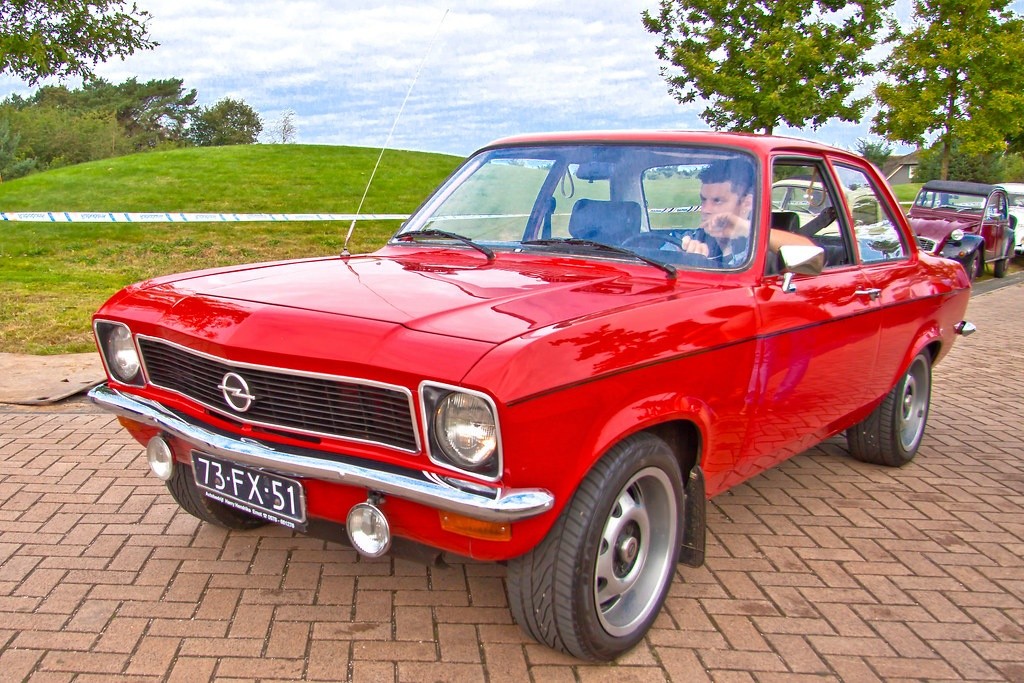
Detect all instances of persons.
[659,159,828,276]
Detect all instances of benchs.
[642,231,844,267]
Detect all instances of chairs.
[769,210,801,272]
[570,198,641,248]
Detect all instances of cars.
[86,127,976,664]
[763,179,889,241]
[901,180,1017,283]
[990,182,1024,256]
